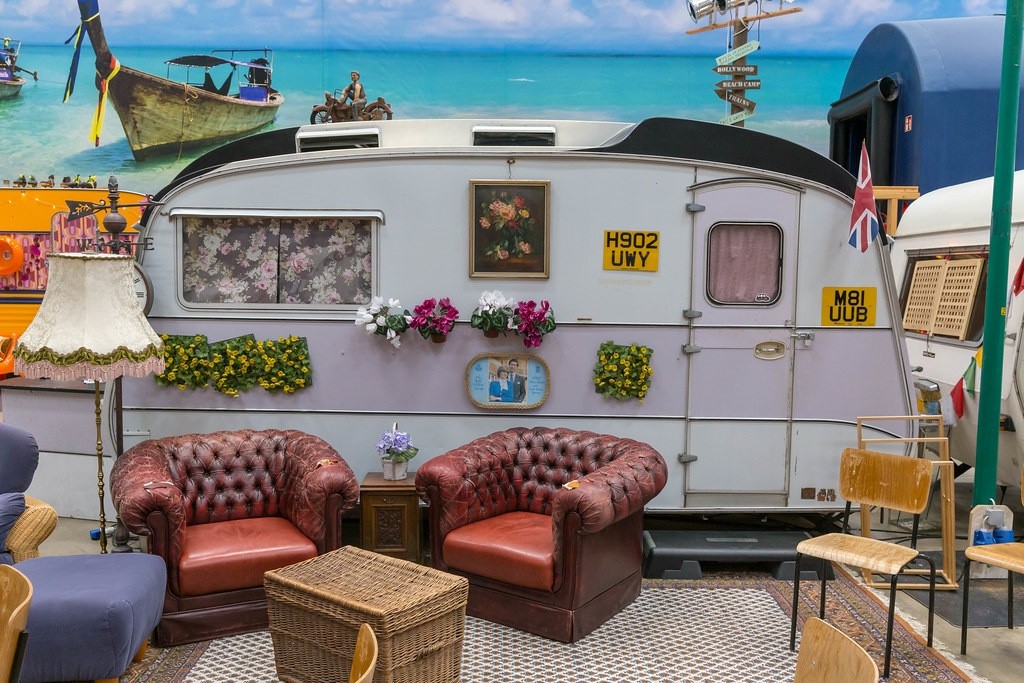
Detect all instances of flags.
[917,258,1024,426]
[849,136,879,252]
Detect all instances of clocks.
[134,261,154,317]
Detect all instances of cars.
[884,163,1020,507]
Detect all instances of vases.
[484,326,499,338]
[431,333,446,343]
[381,423,409,480]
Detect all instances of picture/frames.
[466,353,550,409]
[468,178,551,279]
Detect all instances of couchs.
[0,429,360,683]
[416,425,666,644]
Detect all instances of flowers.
[376,429,419,464]
[153,297,652,403]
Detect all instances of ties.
[510,371,513,382]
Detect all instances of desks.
[0,375,111,458]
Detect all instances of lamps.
[14,252,167,554]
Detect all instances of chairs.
[0,333,17,376]
[349,623,378,683]
[0,564,33,683]
[790,449,1024,682]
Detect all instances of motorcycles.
[310,95,393,126]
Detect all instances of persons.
[336,70,367,122]
[16,174,95,190]
[489,358,526,402]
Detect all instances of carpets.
[116,562,991,683]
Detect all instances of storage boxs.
[263,544,467,683]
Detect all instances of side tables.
[359,472,419,567]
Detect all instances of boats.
[0,35,25,98]
[80,0,289,158]
